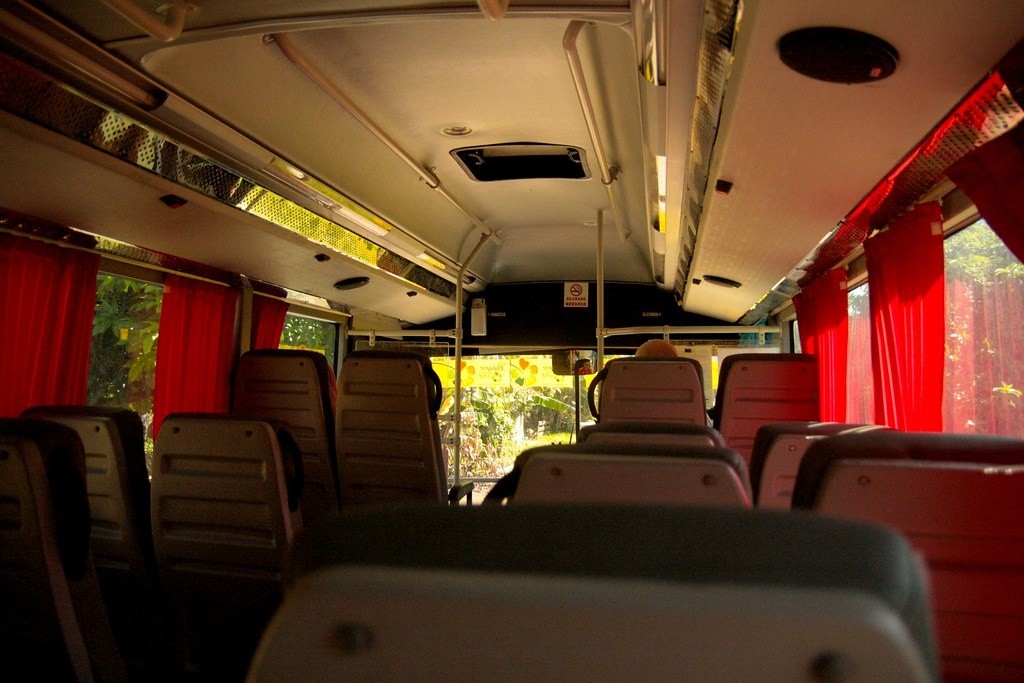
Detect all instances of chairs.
[597,354,708,425]
[580,422,727,449]
[0,417,131,683]
[230,350,340,505]
[225,500,945,683]
[712,352,824,468]
[22,404,154,576]
[754,422,896,501]
[789,433,1024,683]
[148,411,308,672]
[505,444,756,507]
[332,350,477,509]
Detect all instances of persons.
[579,359,592,374]
[635,339,678,359]
